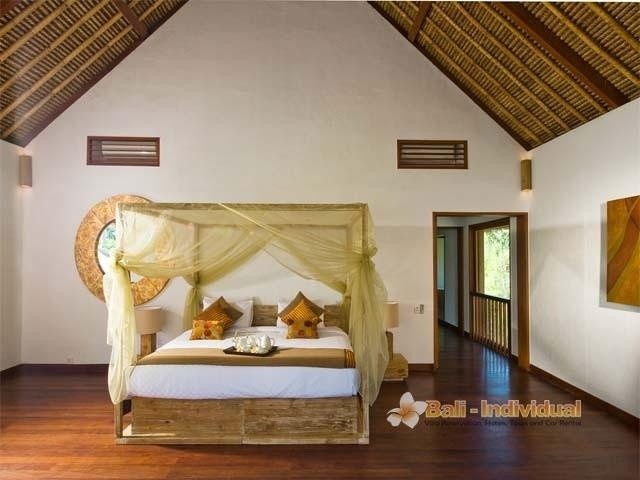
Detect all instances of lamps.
[522,159,532,192]
[21,155,32,189]
[384,299,399,361]
[136,305,165,357]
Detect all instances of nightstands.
[383,352,408,382]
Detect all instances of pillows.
[189,290,327,340]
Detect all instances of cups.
[239,345,266,354]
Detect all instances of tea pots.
[232,329,275,350]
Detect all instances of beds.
[112,203,367,445]
